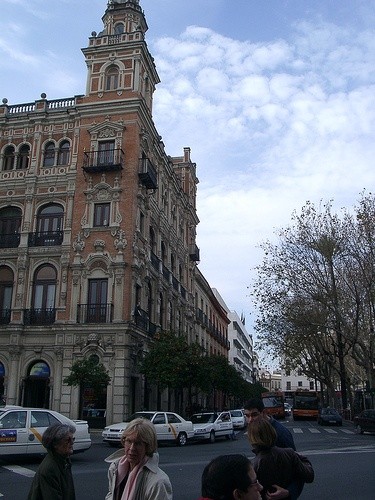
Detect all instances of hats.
[41,422,62,449]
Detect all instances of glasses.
[64,436,75,442]
[249,479,259,487]
[123,437,145,447]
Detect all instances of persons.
[244,398,314,500]
[28,423,76,500]
[202,454,263,500]
[105,417,173,500]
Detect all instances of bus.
[293,387,320,421]
[259,392,286,420]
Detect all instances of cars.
[102,410,195,446]
[319,407,342,427]
[0,407,92,457]
[228,410,246,429]
[353,409,375,435]
[284,402,293,417]
[188,411,235,442]
